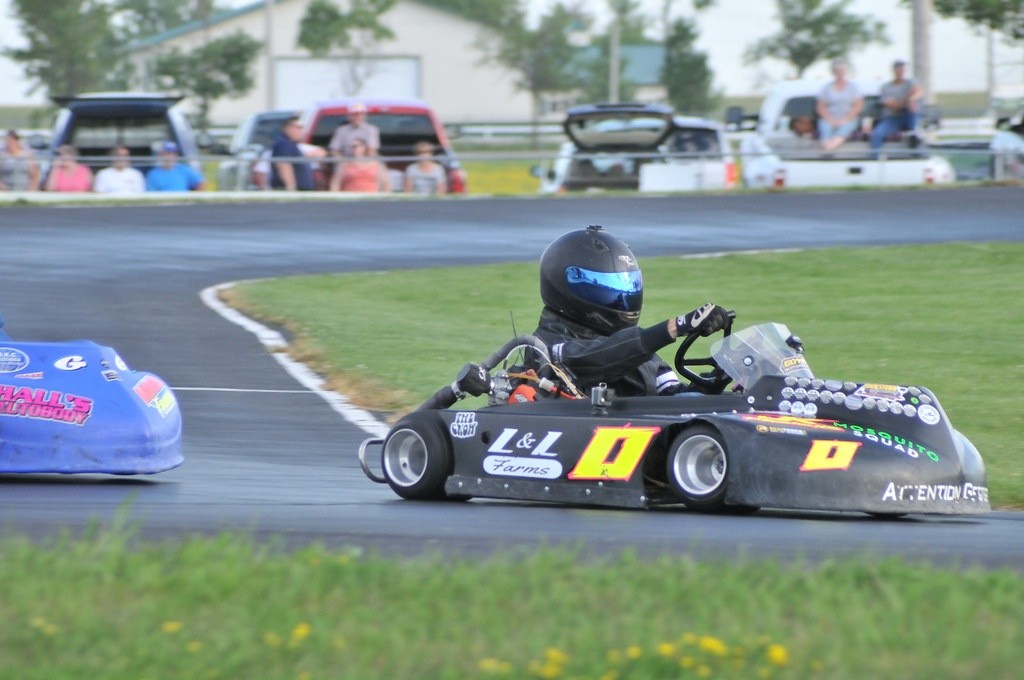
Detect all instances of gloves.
[676,302,729,337]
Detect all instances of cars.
[990,111,1024,181]
[230,110,305,190]
[306,100,466,193]
[30,92,201,191]
[531,102,738,194]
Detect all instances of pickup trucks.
[742,79,948,188]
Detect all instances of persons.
[525,225,729,397]
[0,102,448,194]
[989,118,1024,170]
[787,57,924,159]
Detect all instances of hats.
[348,101,367,113]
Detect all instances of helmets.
[539,224,644,337]
